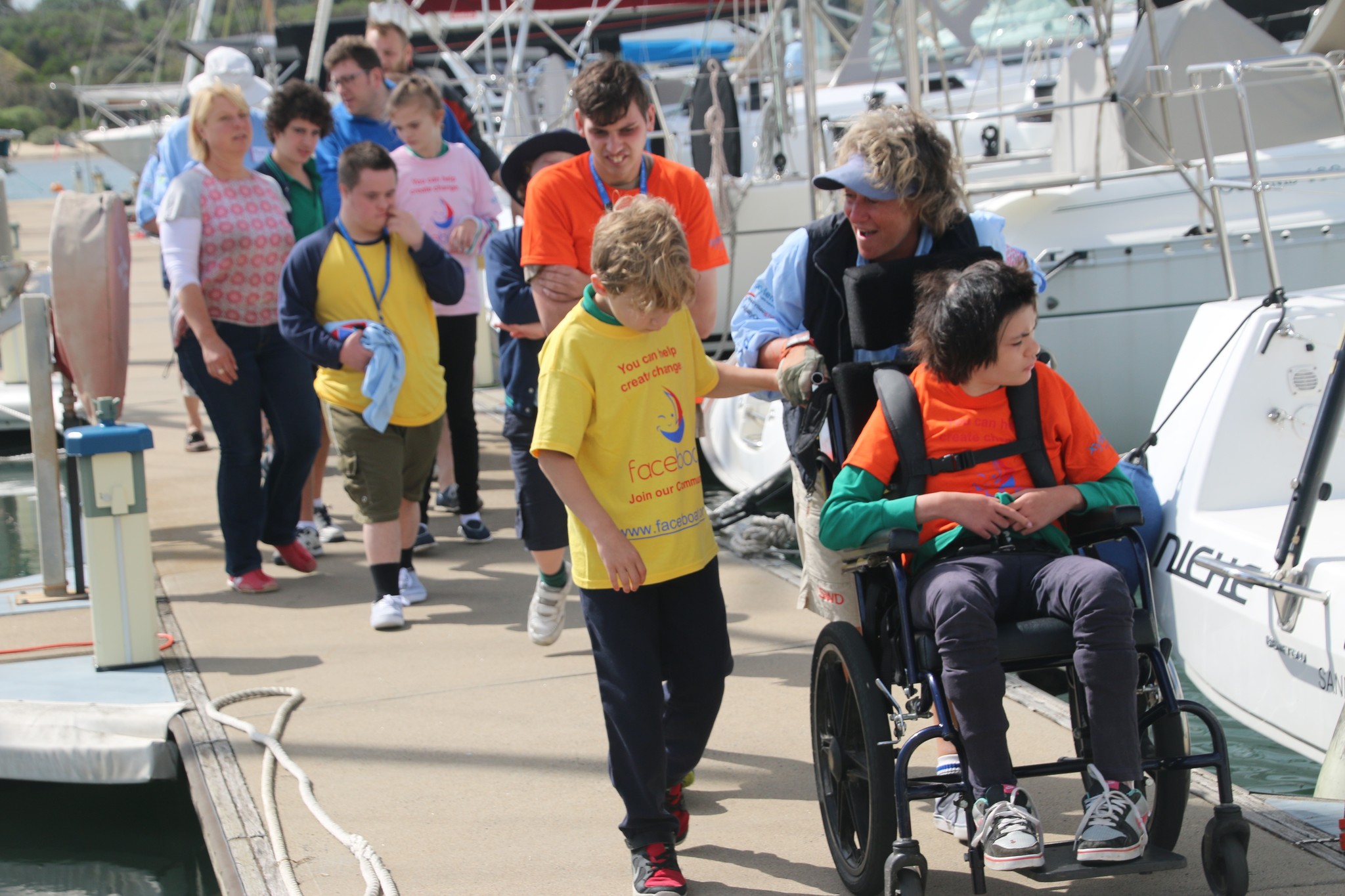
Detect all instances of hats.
[811,152,934,201]
[500,127,591,208]
[187,46,274,106]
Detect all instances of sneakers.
[272,525,324,566]
[1073,763,1149,861]
[370,595,411,629]
[398,567,427,604]
[434,483,483,515]
[527,560,574,646]
[666,783,690,845]
[971,782,1045,872]
[312,503,345,543]
[630,837,687,896]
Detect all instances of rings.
[218,368,225,375]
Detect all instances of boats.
[75,0,1345,762]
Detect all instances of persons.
[156,75,321,593]
[253,76,345,566]
[312,34,481,225]
[484,129,591,646]
[519,60,731,339]
[134,46,275,478]
[278,141,465,630]
[818,260,1149,871]
[528,195,780,896]
[728,103,1047,841]
[365,22,502,193]
[384,74,502,554]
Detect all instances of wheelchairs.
[806,351,1251,895]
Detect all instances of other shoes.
[226,567,279,593]
[274,539,317,573]
[458,512,493,543]
[412,523,439,553]
[681,770,694,789]
[933,786,969,840]
[184,425,208,452]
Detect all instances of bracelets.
[775,330,819,362]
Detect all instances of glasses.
[333,68,371,88]
